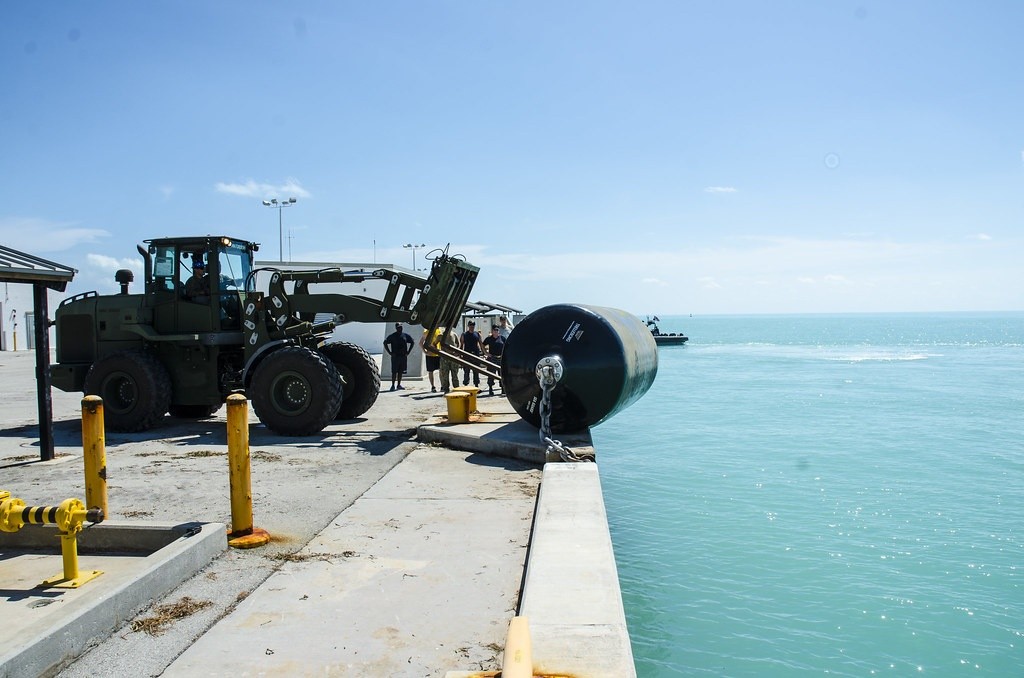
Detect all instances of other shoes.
[397,385,405,389]
[502,392,506,396]
[390,386,395,391]
[431,387,436,393]
[440,387,444,391]
[477,390,482,394]
[489,392,494,396]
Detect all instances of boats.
[644,317,689,345]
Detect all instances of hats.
[396,322,403,327]
[492,324,499,330]
[468,320,475,324]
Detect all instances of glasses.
[469,324,475,327]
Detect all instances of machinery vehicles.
[46,234,482,437]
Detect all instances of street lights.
[402,243,427,273]
[261,195,296,262]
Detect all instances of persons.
[419,327,460,393]
[383,322,415,391]
[185,258,245,331]
[460,316,512,395]
